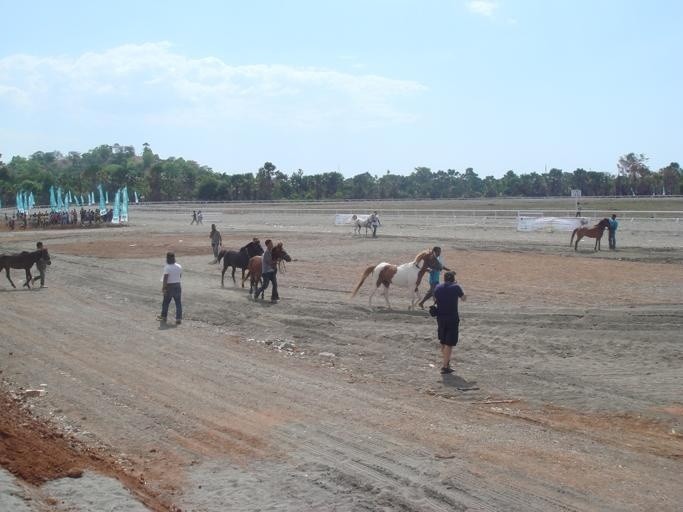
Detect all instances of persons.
[31,242,48,288]
[575,201,582,217]
[372,210,380,237]
[156,251,183,323]
[607,214,619,250]
[4,208,122,231]
[433,271,467,374]
[419,246,457,309]
[210,224,222,257]
[191,209,203,226]
[254,240,281,302]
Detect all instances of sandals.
[440,367,454,373]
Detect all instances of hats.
[444,272,455,283]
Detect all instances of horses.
[351,213,379,237]
[568,218,611,252]
[0,247,52,290]
[350,247,444,312]
[215,241,265,288]
[240,242,292,300]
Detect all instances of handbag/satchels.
[429,305,438,317]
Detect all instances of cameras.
[429,306,436,316]
